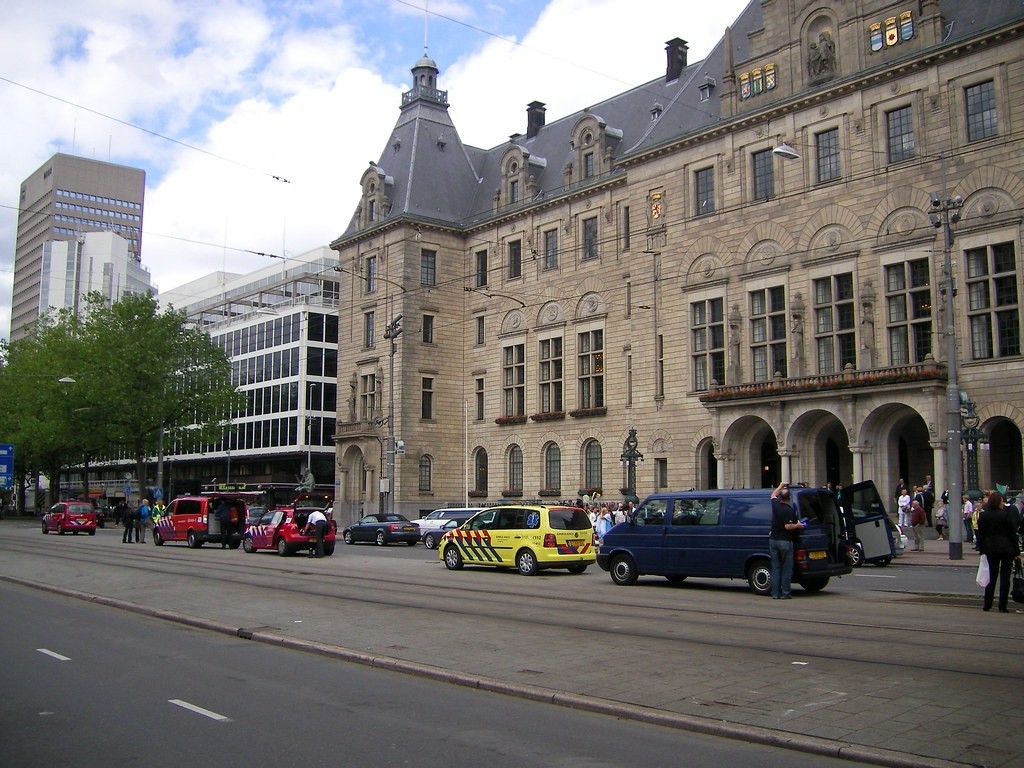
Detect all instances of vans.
[151,491,265,548]
[595,479,898,597]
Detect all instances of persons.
[294,467,315,493]
[908,500,925,551]
[962,494,973,543]
[674,499,697,519]
[1003,496,1024,552]
[972,497,989,551]
[897,488,910,527]
[132,505,141,542]
[114,499,131,528]
[152,498,165,524]
[568,499,636,538]
[822,482,843,492]
[137,498,151,544]
[797,482,809,488]
[894,478,911,517]
[913,474,934,527]
[935,500,945,540]
[941,489,949,529]
[650,504,656,513]
[121,502,136,544]
[214,497,232,549]
[770,482,804,600]
[305,511,328,557]
[977,492,1020,613]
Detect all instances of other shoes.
[773,596,779,598]
[780,596,790,599]
[919,549,924,551]
[911,549,918,551]
[937,537,943,541]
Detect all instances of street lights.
[618,428,646,503]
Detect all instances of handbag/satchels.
[976,554,990,588]
[1010,559,1024,603]
[936,517,943,525]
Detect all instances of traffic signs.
[0,444,14,489]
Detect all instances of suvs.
[410,506,497,537]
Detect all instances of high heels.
[983,607,989,612]
[999,608,1009,613]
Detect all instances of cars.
[94,506,105,528]
[41,501,96,535]
[422,518,485,549]
[438,505,601,576]
[850,523,909,568]
[242,491,336,557]
[343,513,422,547]
